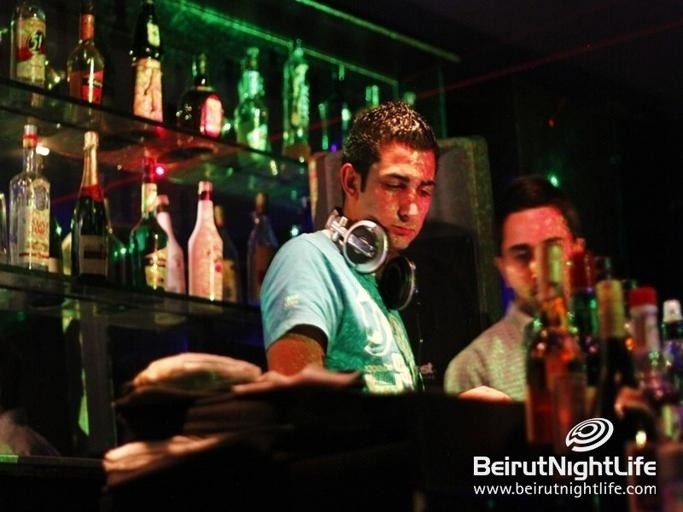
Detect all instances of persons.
[260,100,512,403]
[444,176,587,401]
[1,312,110,459]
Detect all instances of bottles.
[0,0,416,325]
[526,238,683,512]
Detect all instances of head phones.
[327,205,419,312]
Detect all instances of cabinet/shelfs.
[0,76,307,330]
